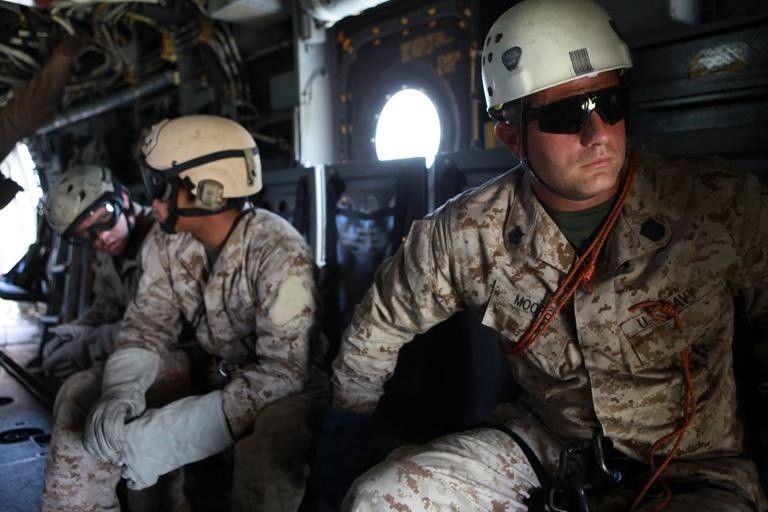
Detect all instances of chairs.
[322,161,427,376]
[624,124,767,189]
[261,163,318,268]
[430,146,520,212]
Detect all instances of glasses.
[69,198,120,246]
[525,81,632,136]
[139,163,167,203]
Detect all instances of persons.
[330,0,768,511]
[40,114,329,510]
[40,163,154,383]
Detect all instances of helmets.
[46,164,120,245]
[142,116,263,200]
[481,1,633,112]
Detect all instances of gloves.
[84,348,159,463]
[42,323,121,384]
[118,390,236,492]
